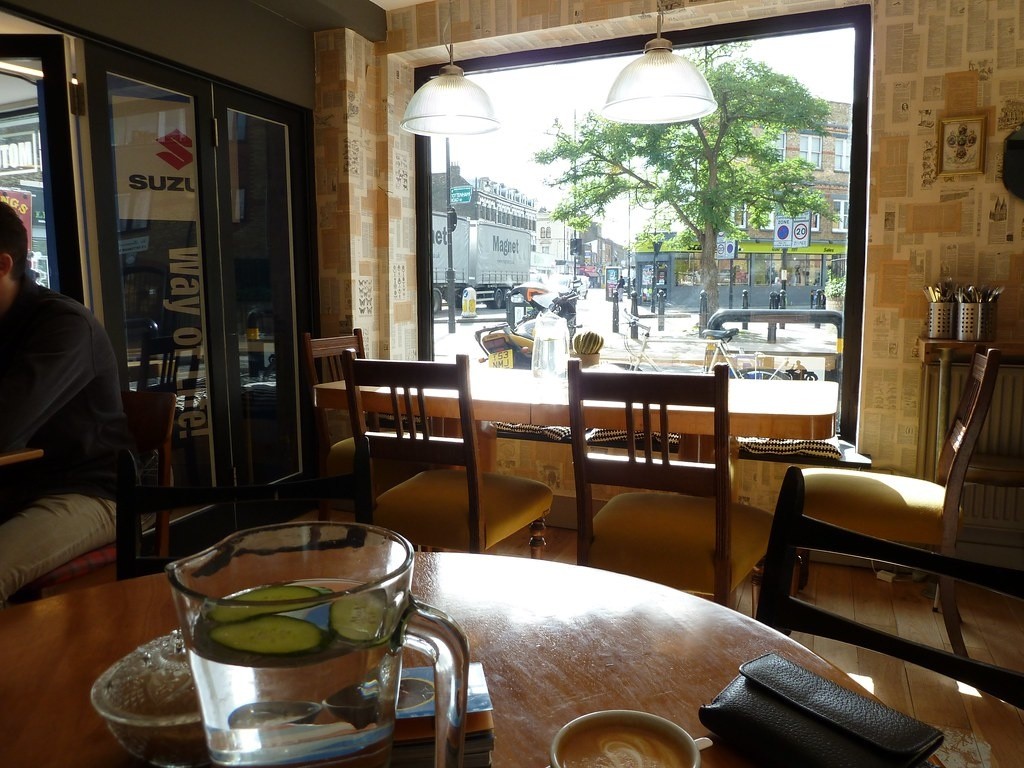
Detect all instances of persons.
[617,276,625,301]
[0,201,157,611]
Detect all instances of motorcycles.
[783,370,818,381]
[474,292,583,368]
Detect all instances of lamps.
[600,0,718,124]
[394,0,499,137]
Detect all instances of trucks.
[431,209,532,315]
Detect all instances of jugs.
[531,320,567,379]
[166,521,469,768]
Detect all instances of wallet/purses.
[699,650,945,768]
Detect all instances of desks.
[314,367,840,500]
[0,549,946,767]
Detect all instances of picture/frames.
[934,113,987,177]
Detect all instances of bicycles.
[618,307,794,380]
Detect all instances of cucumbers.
[205,585,391,659]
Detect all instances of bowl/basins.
[91,629,214,768]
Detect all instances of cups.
[545,710,701,768]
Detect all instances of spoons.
[955,285,1004,303]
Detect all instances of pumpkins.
[574,331,605,354]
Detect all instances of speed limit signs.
[794,224,808,240]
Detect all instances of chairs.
[339,347,552,562]
[565,357,772,614]
[788,345,1002,656]
[21,388,176,599]
[300,326,390,520]
